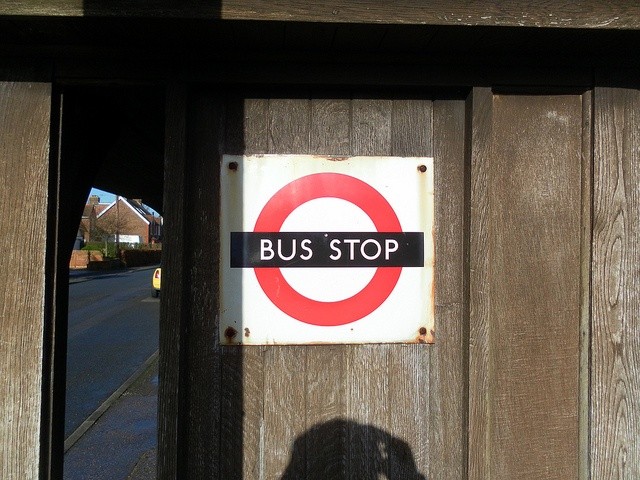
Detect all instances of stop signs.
[217,155,435,347]
